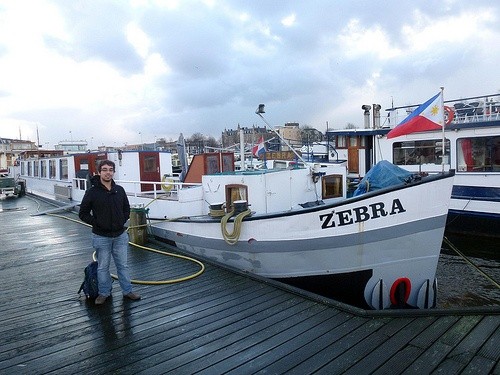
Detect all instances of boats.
[119,132,331,174]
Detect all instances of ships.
[14,144,456,308]
[178,93,500,238]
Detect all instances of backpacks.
[78,261,114,297]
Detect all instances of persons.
[79,160,143,305]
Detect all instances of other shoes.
[94,293,141,304]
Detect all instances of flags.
[386,91,443,139]
[251,136,267,160]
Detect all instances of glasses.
[100,169,114,172]
